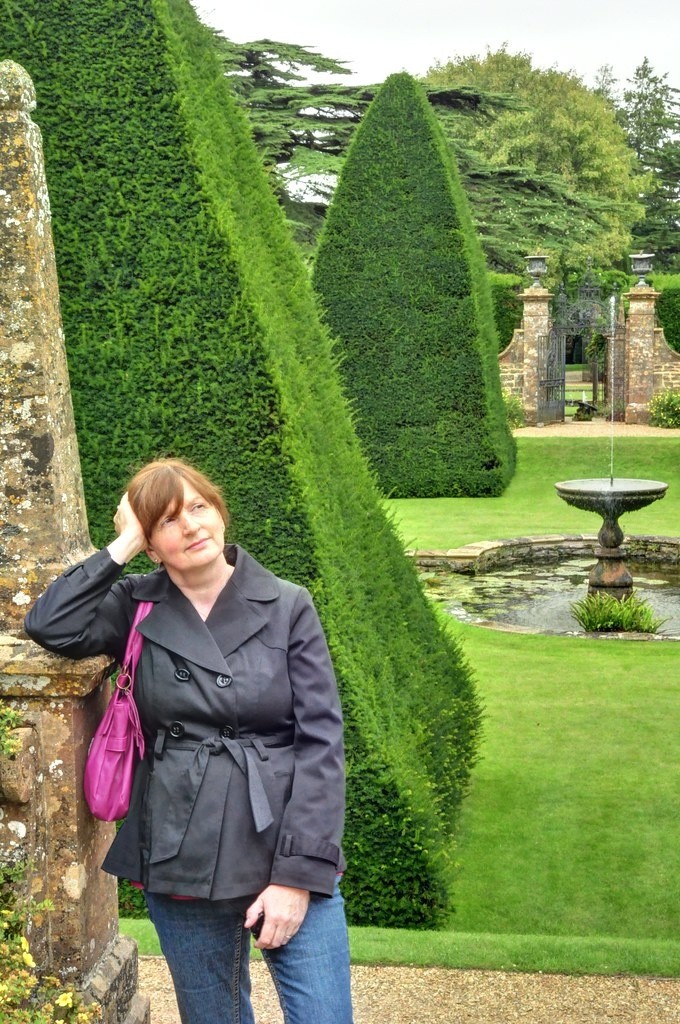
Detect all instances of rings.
[284,934,293,941]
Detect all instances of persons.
[23,459,356,1024]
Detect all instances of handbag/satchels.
[82,672,145,824]
